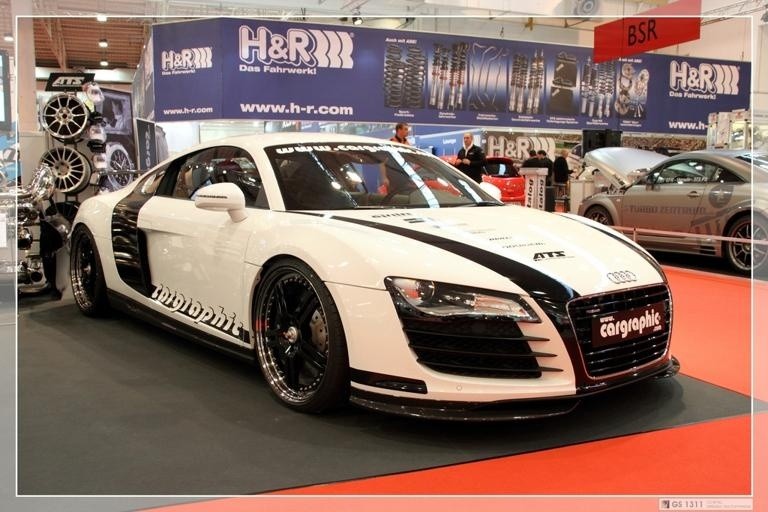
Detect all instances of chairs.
[194,161,260,207]
[280,163,354,210]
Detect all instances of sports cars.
[68,132,680,423]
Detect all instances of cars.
[577,139,767,275]
[377,155,527,208]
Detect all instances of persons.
[552,148,577,212]
[520,149,541,178]
[453,132,485,185]
[536,149,554,187]
[385,121,416,194]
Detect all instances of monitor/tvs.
[581,128,623,158]
